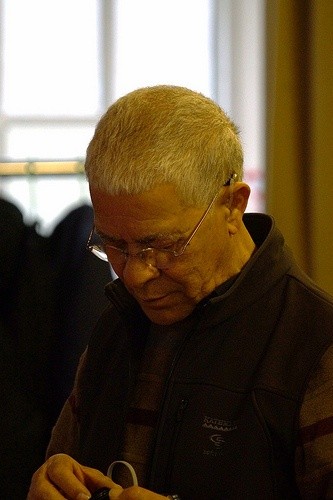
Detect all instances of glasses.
[86,173,237,269]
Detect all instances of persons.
[22,84,333,499]
